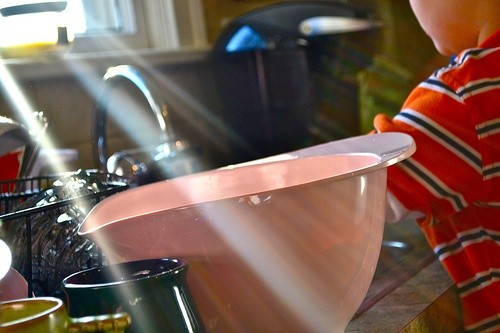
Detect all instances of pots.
[0,297,131,333]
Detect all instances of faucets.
[106,151,148,176]
[91,64,203,170]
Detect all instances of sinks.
[350,230,440,317]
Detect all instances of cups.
[61,257,206,333]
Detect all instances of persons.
[368,0,500,332]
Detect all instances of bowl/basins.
[77,132,417,333]
